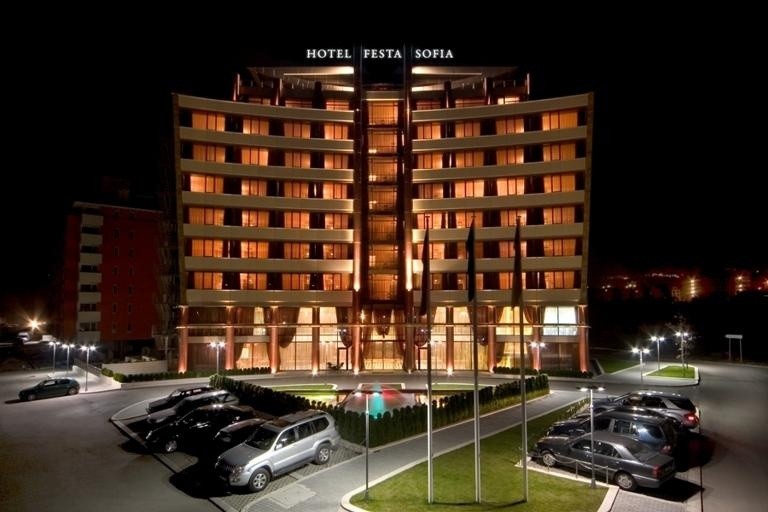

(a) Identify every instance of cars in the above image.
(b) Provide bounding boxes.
[528,390,699,492]
[144,385,341,493]
[19,379,79,401]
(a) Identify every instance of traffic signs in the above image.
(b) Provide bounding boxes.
[725,335,742,339]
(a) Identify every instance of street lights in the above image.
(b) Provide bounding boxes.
[675,332,687,367]
[631,347,649,384]
[62,344,74,376]
[650,336,664,370]
[210,342,225,374]
[81,345,95,391]
[48,342,60,375]
[530,342,544,372]
[351,390,382,503]
